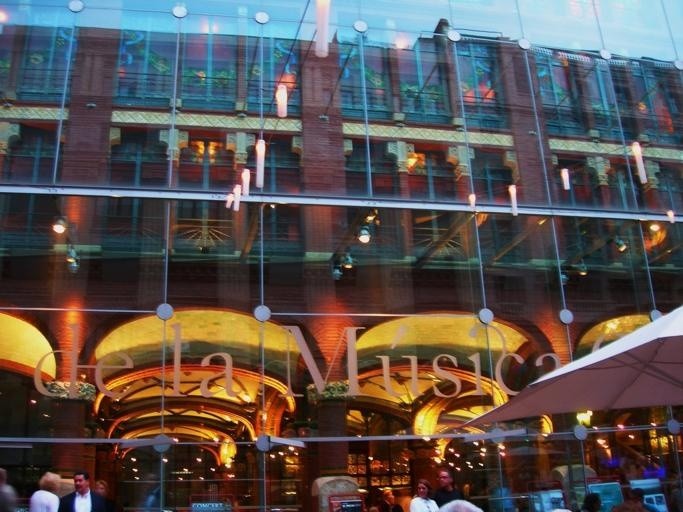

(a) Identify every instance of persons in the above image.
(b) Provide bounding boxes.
[0,465,126,512]
[579,491,602,512]
[432,468,462,507]
[409,480,439,512]
[611,488,656,512]
[137,473,165,512]
[380,489,400,512]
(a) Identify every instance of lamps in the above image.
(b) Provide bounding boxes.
[558,236,629,290]
[50,218,81,277]
[328,220,370,283]
